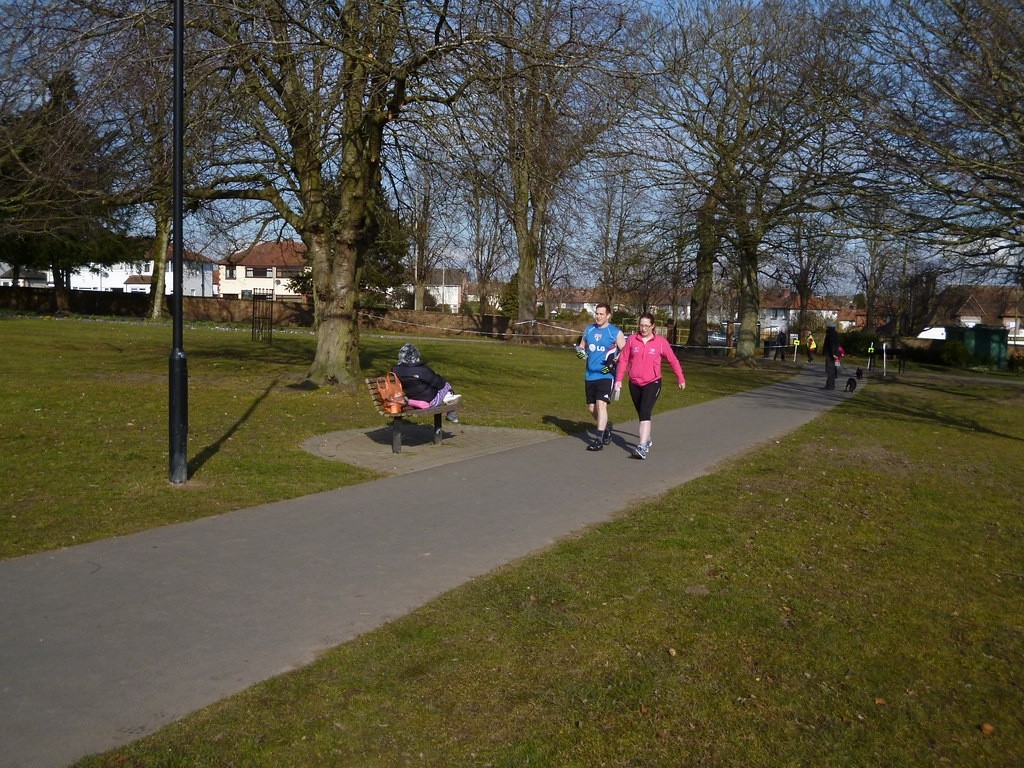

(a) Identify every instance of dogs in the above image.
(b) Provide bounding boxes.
[845,366,864,393]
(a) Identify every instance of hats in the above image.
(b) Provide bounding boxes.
[398,343,421,364]
[806,330,811,334]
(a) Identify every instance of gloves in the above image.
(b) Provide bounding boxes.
[576,348,585,359]
[601,361,614,374]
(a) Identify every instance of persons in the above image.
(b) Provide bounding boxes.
[391,344,458,423]
[576,302,626,451]
[805,331,817,363]
[820,322,844,390]
[774,330,787,361]
[613,313,686,459]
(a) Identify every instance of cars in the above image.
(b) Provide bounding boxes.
[708,330,739,347]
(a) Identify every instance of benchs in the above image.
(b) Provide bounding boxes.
[364,374,460,453]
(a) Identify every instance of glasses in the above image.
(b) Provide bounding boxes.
[639,323,653,328]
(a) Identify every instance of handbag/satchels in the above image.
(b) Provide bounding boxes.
[376,372,408,414]
[835,359,841,367]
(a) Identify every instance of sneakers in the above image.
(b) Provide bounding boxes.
[442,389,462,405]
[587,440,603,451]
[446,411,459,422]
[603,422,614,445]
[646,436,652,452]
[632,444,646,459]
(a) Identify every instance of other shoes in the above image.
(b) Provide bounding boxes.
[811,360,815,364]
[805,360,811,364]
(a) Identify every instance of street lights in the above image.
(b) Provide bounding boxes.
[442,266,446,303]
[414,185,419,286]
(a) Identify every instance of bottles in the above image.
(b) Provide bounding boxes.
[615,384,620,401]
[602,367,610,373]
[574,344,588,360]
